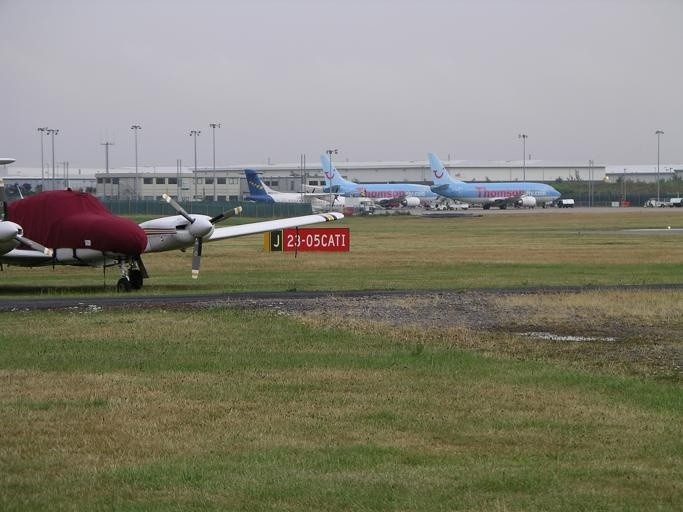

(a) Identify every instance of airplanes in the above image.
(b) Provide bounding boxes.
[0,158,345,294]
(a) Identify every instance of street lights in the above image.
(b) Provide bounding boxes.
[37,127,59,191]
[518,133,529,182]
[209,123,221,203]
[326,149,337,213]
[99,141,115,174]
[130,124,142,173]
[655,130,664,201]
[188,129,201,195]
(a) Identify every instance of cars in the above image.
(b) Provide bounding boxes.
[644,198,683,208]
